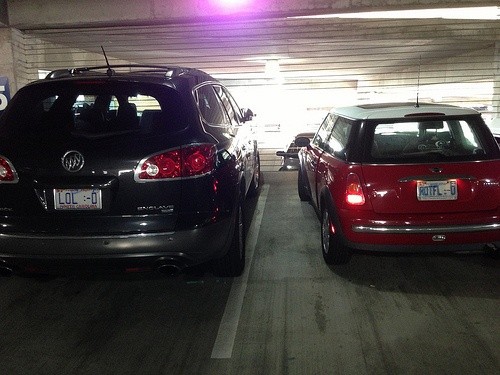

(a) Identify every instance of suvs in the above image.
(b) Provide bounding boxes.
[0,64,261,280]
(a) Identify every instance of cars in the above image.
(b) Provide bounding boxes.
[275,133,319,170]
[294,101,500,266]
